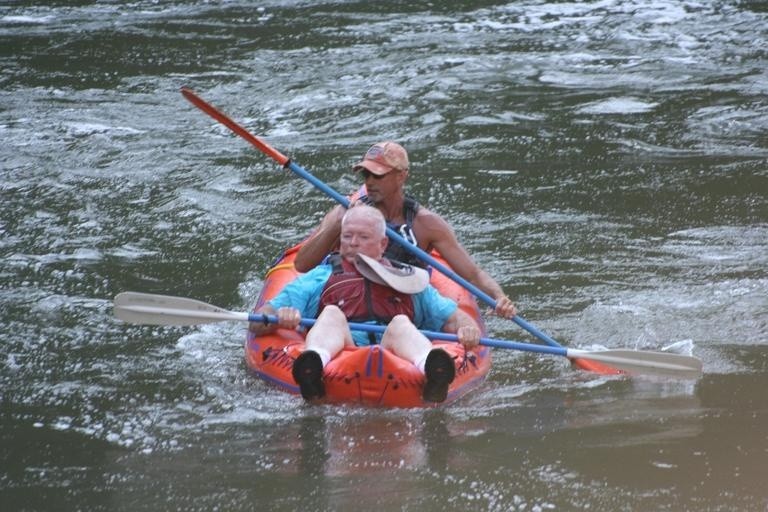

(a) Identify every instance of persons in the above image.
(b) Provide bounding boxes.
[294,141,520,320]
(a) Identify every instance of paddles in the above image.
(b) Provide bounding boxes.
[178,89,635,375]
[114,293,703,380]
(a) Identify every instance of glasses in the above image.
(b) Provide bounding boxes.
[360,170,386,179]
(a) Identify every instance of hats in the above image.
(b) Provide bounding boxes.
[352,140,409,176]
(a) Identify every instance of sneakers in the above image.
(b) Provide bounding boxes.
[421,346,457,404]
[292,349,327,404]
